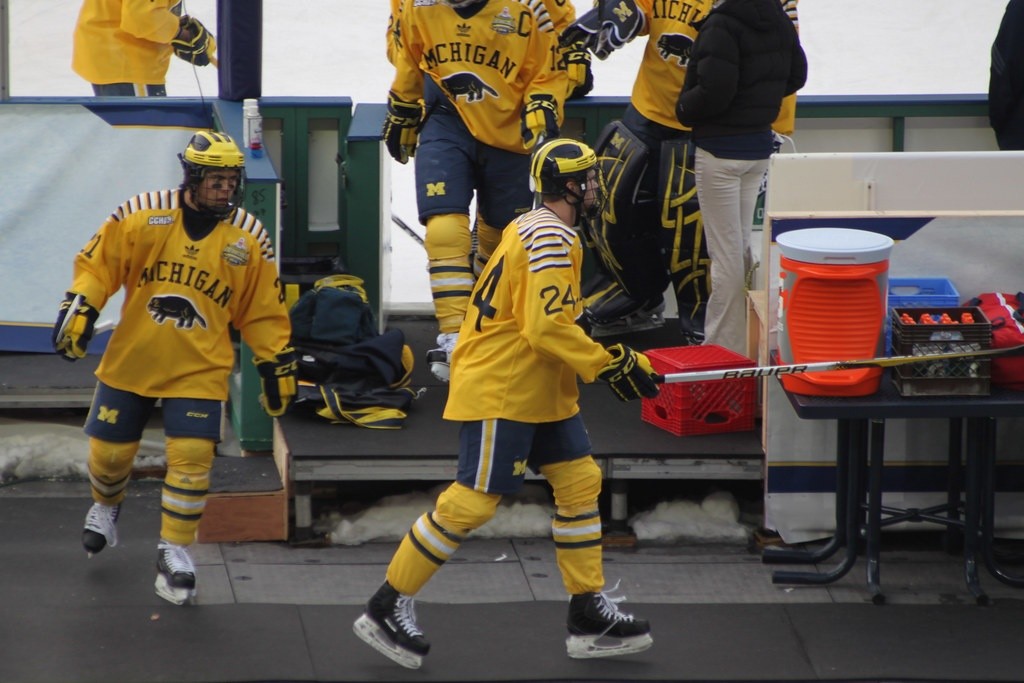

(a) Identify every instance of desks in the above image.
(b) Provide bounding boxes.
[762,349,1024,605]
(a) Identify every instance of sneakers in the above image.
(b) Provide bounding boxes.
[583,289,666,337]
[426,330,464,382]
[153,534,198,608]
[350,574,431,670]
[684,329,705,346]
[563,583,653,660]
[81,501,120,559]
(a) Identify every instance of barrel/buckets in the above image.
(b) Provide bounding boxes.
[775,227,895,397]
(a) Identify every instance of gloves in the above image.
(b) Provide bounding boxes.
[253,348,299,420]
[594,346,659,402]
[52,292,100,363]
[517,99,556,150]
[168,12,217,67]
[560,50,593,101]
[382,91,422,166]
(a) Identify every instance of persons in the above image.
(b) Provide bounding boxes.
[352,138,661,668]
[675,0,808,357]
[560,0,799,341]
[50,132,297,604]
[384,0,593,382]
[70,0,216,96]
[987,0,1024,150]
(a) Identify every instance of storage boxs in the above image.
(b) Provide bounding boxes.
[892,305,992,397]
[641,343,758,437]
[887,276,960,359]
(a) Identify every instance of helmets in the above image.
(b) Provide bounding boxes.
[528,136,608,221]
[178,131,247,221]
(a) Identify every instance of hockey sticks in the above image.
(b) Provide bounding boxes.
[646,331,1022,385]
[208,52,426,248]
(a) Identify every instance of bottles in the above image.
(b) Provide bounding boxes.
[241,97,266,159]
